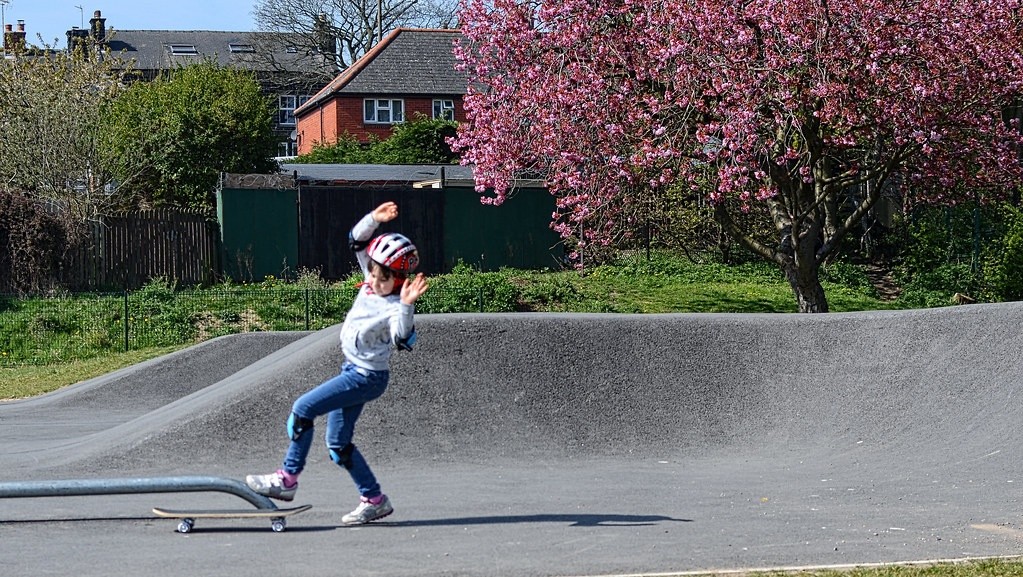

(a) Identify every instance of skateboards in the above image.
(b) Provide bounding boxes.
[152,503,313,534]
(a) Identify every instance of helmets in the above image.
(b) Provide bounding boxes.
[367,232,421,273]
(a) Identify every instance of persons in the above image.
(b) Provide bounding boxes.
[251,199,425,523]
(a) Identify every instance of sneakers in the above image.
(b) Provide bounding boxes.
[245,471,298,502]
[341,495,392,526]
